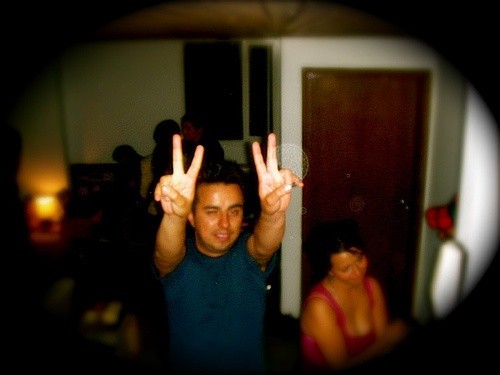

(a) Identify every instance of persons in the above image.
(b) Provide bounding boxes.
[300,236,408,370]
[0,109,305,375]
